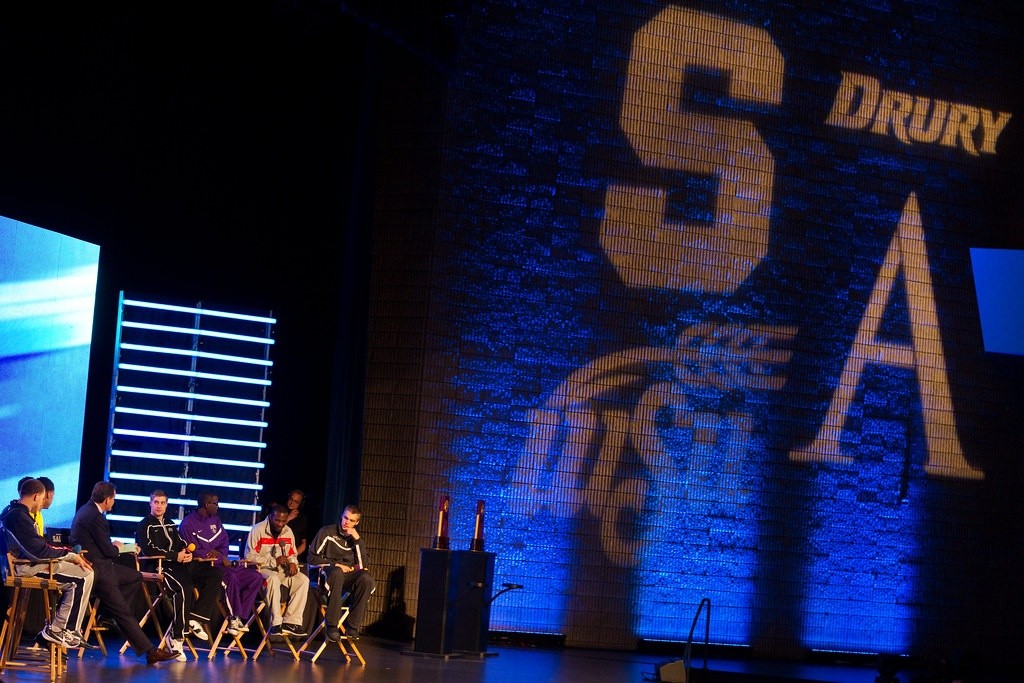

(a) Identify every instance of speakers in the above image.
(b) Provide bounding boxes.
[655,660,686,683]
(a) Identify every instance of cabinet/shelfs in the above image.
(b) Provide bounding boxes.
[413,548,498,656]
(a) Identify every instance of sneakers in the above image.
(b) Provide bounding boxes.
[42,625,80,649]
[324,617,340,643]
[165,632,188,662]
[235,616,249,632]
[226,617,238,634]
[188,620,208,641]
[282,623,309,637]
[269,625,281,635]
[345,623,359,640]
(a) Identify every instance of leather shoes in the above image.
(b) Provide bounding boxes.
[97,614,121,635]
[73,633,94,651]
[34,631,69,661]
[146,648,181,665]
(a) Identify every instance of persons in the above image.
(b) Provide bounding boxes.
[137,490,263,662]
[243,488,374,642]
[0,476,95,649]
[69,481,182,664]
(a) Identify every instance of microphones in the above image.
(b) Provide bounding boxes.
[73,544,82,553]
[185,543,196,554]
[351,565,360,571]
[279,540,287,568]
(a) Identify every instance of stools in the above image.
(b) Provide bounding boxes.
[0,549,367,681]
